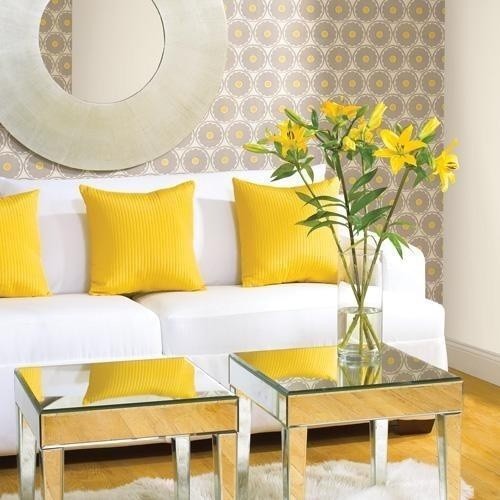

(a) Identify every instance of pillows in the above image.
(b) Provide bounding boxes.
[82,359,197,405]
[0,189,52,297]
[78,180,206,295]
[239,346,339,383]
[17,367,44,403]
[232,177,342,287]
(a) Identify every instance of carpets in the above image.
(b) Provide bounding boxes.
[0,458,474,499]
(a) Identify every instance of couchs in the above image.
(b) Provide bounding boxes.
[0,162,448,457]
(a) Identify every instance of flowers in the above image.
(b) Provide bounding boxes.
[243,102,459,353]
